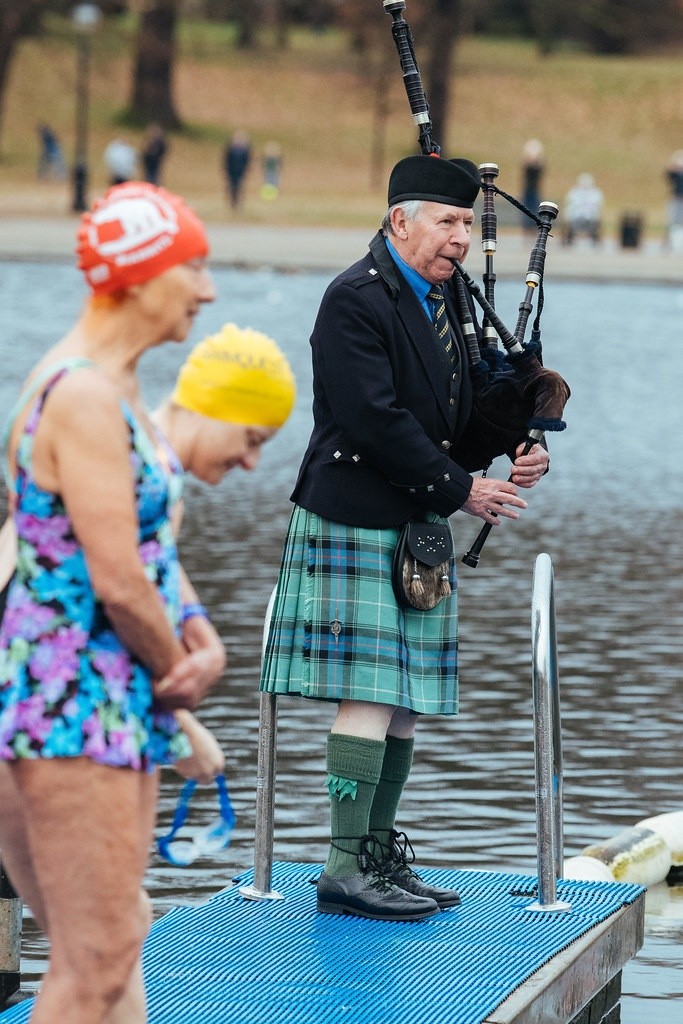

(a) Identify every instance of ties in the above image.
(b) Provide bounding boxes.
[428,284,457,366]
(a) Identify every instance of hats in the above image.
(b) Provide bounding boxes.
[75,181,212,298]
[387,155,482,208]
[171,323,297,428]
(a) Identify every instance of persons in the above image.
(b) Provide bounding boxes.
[25,108,256,211]
[520,142,683,256]
[259,153,551,920]
[0,179,296,1024]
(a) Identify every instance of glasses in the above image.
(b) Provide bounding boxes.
[159,822,230,863]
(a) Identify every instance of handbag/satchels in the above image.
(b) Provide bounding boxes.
[391,519,453,612]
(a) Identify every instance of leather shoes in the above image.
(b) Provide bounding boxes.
[316,833,440,921]
[374,827,462,907]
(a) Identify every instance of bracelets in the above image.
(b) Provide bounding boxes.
[179,603,211,627]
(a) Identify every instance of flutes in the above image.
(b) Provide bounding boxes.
[387,1,571,568]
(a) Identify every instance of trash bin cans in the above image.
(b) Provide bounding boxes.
[621,216,640,246]
